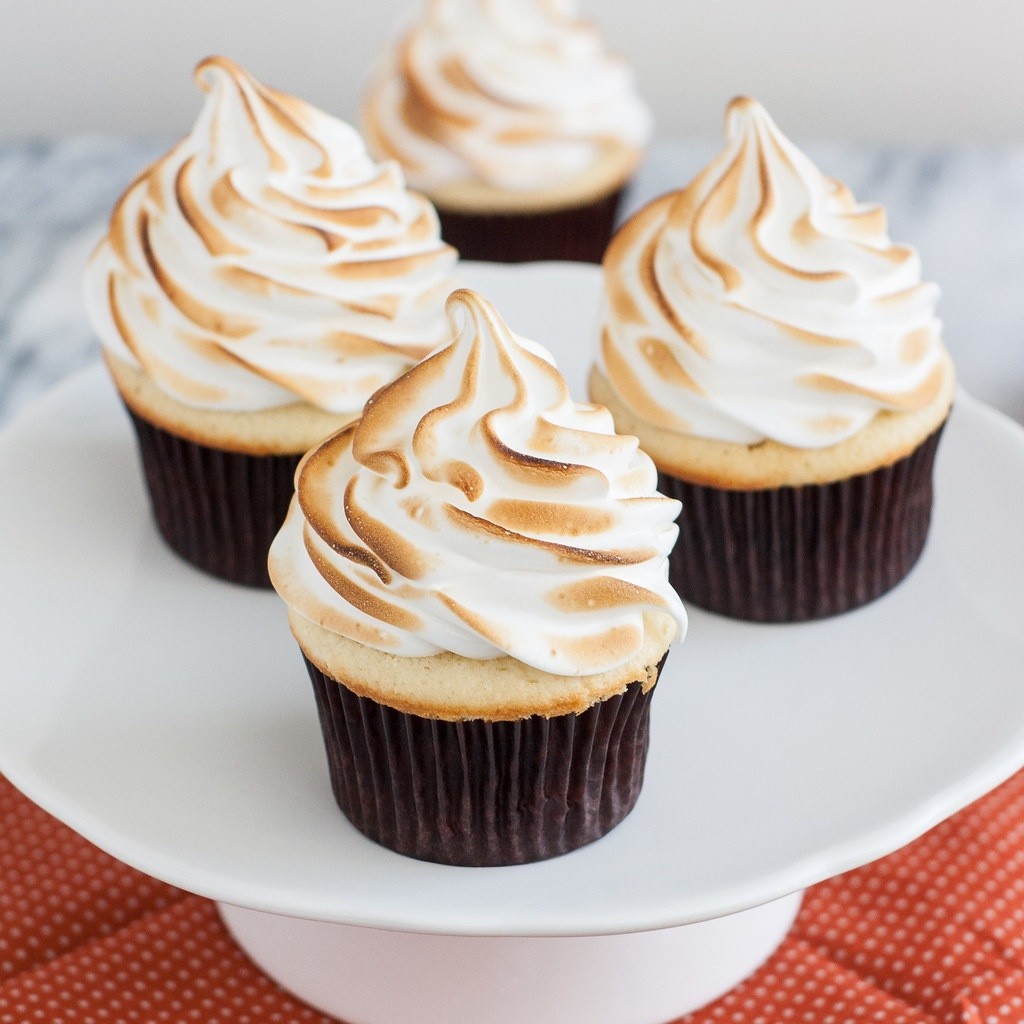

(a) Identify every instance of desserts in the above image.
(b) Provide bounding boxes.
[588,98,957,620]
[85,57,463,591]
[266,291,690,867]
[364,1,652,263]
